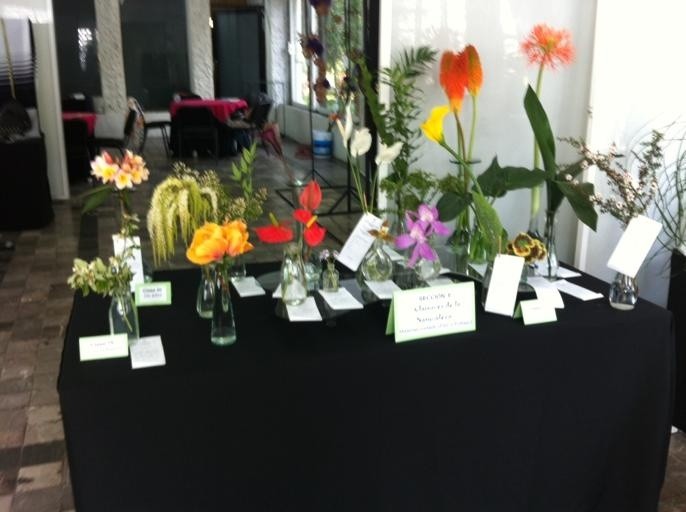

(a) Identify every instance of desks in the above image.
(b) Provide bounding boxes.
[60,109,97,159]
[56,256,676,512]
[170,97,251,160]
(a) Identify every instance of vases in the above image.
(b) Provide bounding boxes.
[411,250,443,282]
[110,230,145,294]
[360,218,395,282]
[322,262,340,293]
[225,250,247,285]
[195,262,216,319]
[281,241,308,307]
[210,259,237,347]
[446,228,472,247]
[109,288,141,348]
[480,263,492,308]
[608,271,640,311]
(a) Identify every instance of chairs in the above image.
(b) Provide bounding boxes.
[81,110,136,190]
[124,95,172,156]
[245,94,285,157]
[172,89,201,101]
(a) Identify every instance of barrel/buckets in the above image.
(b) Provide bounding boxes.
[313,129,332,160]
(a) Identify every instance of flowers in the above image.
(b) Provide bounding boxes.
[418,104,504,263]
[145,160,220,307]
[436,43,483,234]
[298,0,342,108]
[65,210,142,334]
[255,179,327,283]
[318,246,342,291]
[552,127,663,226]
[198,138,268,267]
[72,146,151,234]
[326,68,352,133]
[392,203,455,269]
[520,25,576,222]
[504,230,549,284]
[335,105,405,281]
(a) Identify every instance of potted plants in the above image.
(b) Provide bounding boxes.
[470,81,599,283]
